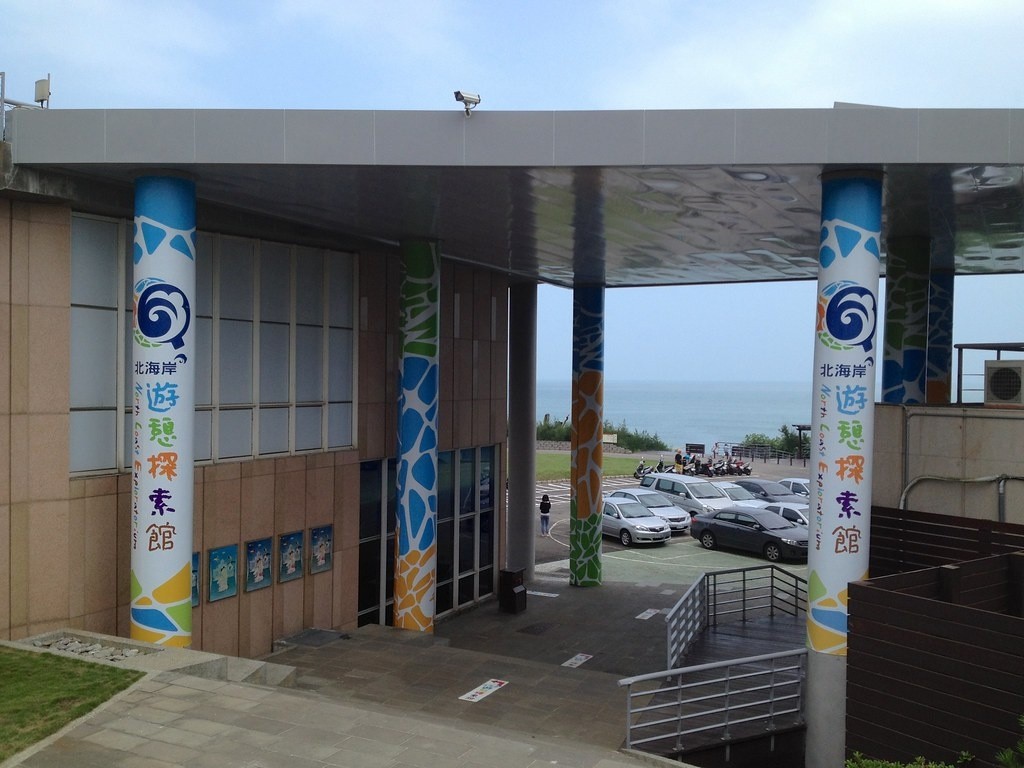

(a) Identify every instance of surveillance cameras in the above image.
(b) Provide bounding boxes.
[454,91,481,109]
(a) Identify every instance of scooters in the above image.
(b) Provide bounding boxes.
[632,453,752,477]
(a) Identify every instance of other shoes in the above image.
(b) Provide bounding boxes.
[547,534,549,536]
[542,534,544,536]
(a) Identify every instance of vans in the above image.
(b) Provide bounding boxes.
[639,472,735,518]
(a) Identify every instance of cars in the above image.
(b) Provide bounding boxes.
[778,478,810,499]
[690,505,808,563]
[601,497,672,547]
[739,479,810,506]
[611,488,690,532]
[710,481,769,509]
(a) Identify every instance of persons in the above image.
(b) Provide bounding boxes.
[540,494,552,537]
[674,449,691,475]
[723,444,729,459]
[713,443,720,459]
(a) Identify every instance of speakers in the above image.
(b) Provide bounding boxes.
[35,79,49,103]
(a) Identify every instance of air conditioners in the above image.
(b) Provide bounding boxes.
[984,360,1024,409]
